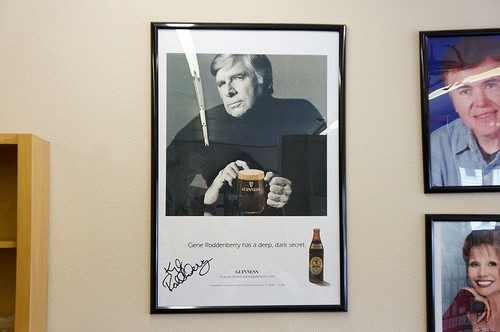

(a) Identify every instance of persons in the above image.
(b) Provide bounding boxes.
[165,54,327,216]
[429,37,500,186]
[442,225,500,332]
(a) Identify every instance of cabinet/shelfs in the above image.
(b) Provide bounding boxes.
[1,132,50,331]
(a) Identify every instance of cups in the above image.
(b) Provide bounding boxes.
[236,170,280,215]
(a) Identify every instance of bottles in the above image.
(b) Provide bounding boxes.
[309,229,324,284]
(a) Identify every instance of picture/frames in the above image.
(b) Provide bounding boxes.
[423,213,499,332]
[150,21,349,314]
[418,26,500,195]
